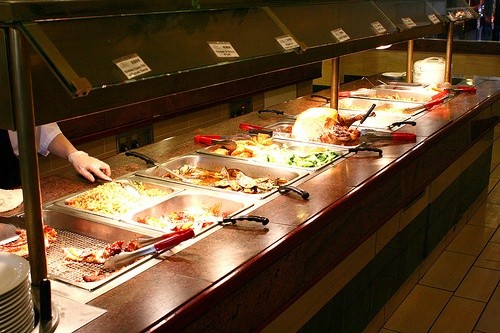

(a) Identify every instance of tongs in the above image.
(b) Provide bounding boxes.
[443,84,477,95]
[193,132,251,152]
[359,127,416,143]
[240,121,294,139]
[103,228,196,273]
[403,94,451,114]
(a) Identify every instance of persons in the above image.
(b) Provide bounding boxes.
[1,122,112,188]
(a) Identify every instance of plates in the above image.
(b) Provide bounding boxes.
[382,71,406,80]
[0,249,36,333]
